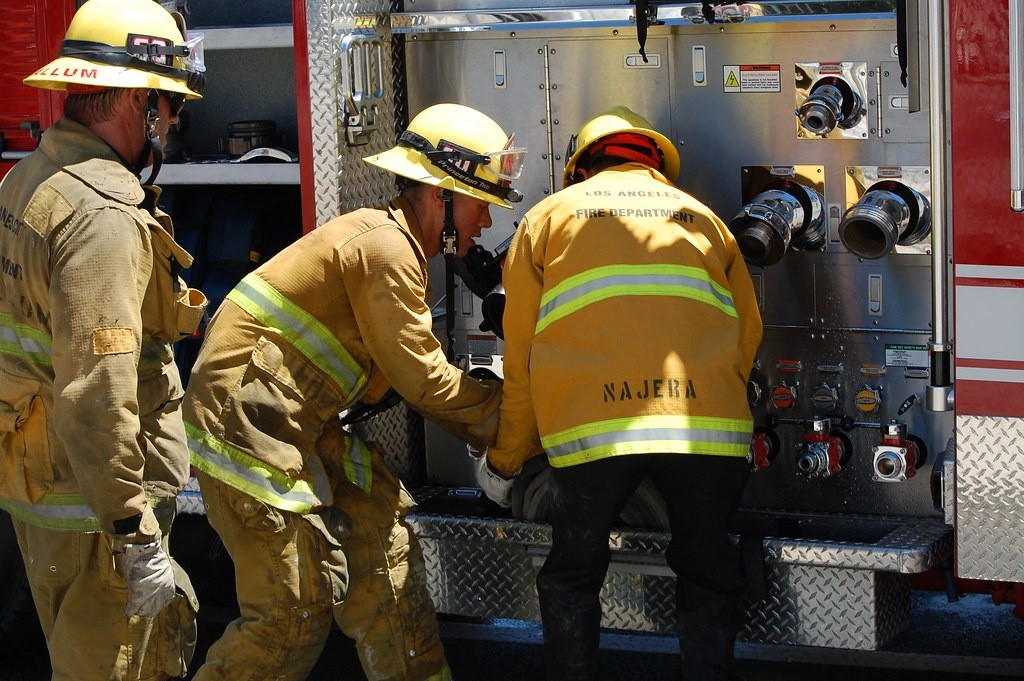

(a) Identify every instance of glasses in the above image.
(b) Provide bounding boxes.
[181,31,207,72]
[158,90,186,118]
[480,147,529,181]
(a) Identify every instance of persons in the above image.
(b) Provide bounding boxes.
[0,0,209,681]
[182,104,519,680]
[474,104,762,681]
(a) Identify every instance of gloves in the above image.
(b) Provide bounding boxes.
[466,444,483,461]
[475,446,523,510]
[122,536,175,617]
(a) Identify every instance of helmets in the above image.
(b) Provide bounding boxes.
[362,103,514,211]
[563,105,681,188]
[23,0,203,99]
